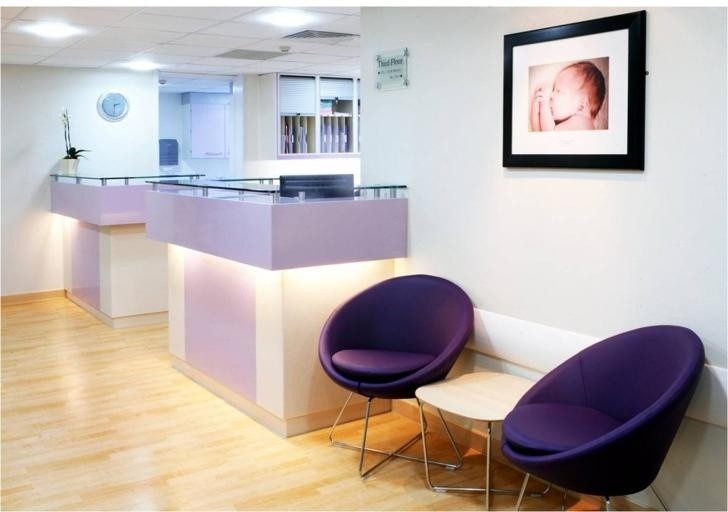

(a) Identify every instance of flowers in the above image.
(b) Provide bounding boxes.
[56,106,92,160]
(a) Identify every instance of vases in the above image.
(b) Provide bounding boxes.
[57,158,81,176]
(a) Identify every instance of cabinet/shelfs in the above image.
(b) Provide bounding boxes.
[256,72,360,160]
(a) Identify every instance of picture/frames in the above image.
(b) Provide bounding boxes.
[502,9,646,171]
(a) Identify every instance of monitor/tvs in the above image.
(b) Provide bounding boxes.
[279,173,355,198]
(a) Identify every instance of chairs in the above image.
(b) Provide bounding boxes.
[317,273,474,478]
[501,325,707,512]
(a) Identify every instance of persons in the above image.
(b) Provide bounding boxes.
[529,61,606,132]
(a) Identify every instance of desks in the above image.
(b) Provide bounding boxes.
[416,370,552,509]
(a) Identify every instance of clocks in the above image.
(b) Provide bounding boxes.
[96,90,129,123]
